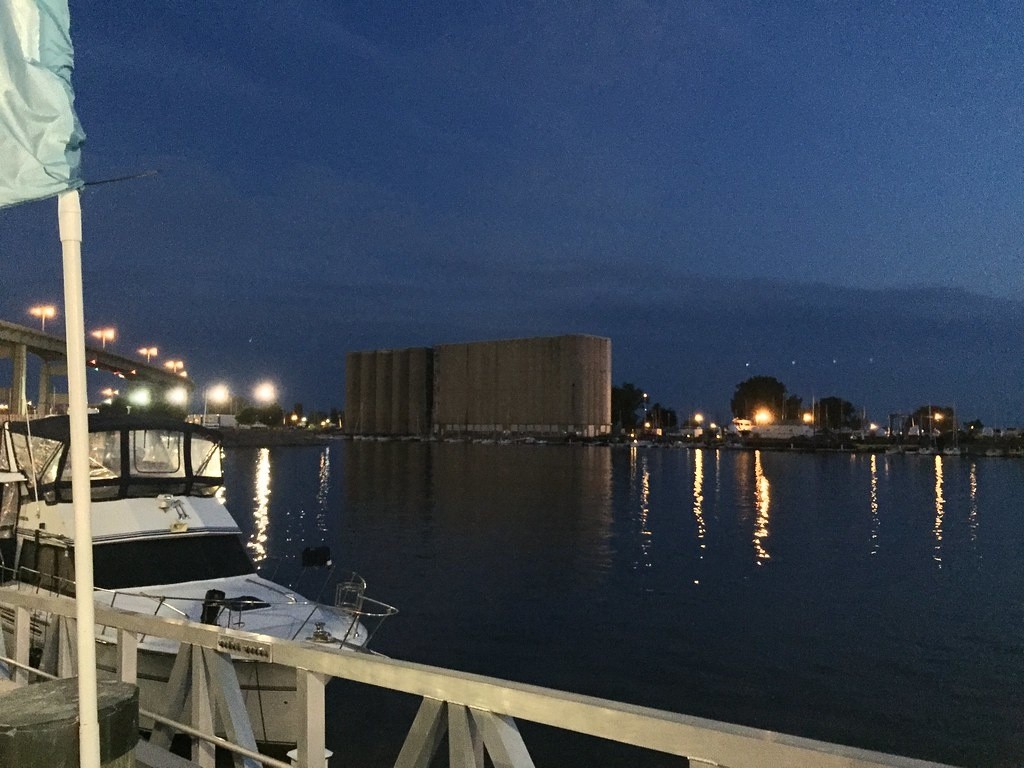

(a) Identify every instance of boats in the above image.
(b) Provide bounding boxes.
[1,399,401,751]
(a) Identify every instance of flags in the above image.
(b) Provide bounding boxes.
[0,0,81,206]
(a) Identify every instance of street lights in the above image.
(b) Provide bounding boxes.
[138,347,158,366]
[164,360,184,376]
[92,327,115,352]
[31,305,56,332]
